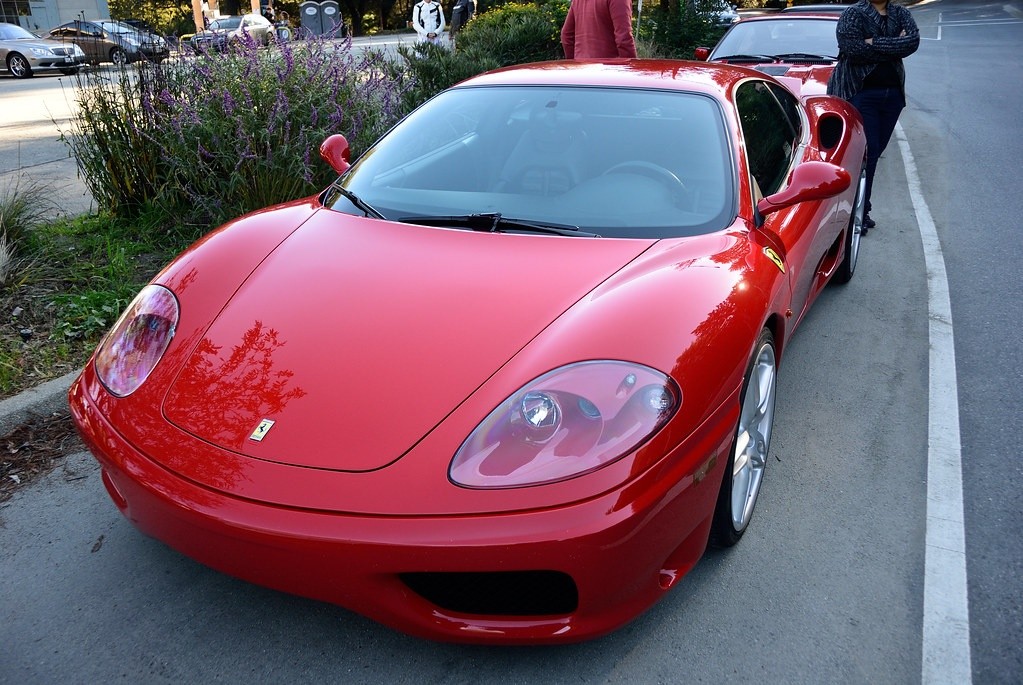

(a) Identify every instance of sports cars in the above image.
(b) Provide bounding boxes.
[66,57,870,649]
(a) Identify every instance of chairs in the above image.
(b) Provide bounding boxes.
[495,103,589,192]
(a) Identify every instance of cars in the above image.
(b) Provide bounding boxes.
[40,19,170,67]
[0,22,86,79]
[189,13,276,53]
[692,2,852,98]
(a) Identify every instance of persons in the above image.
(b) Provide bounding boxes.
[264,8,275,24]
[828,0,920,237]
[449,0,477,39]
[651,122,763,215]
[561,0,639,60]
[412,0,446,61]
